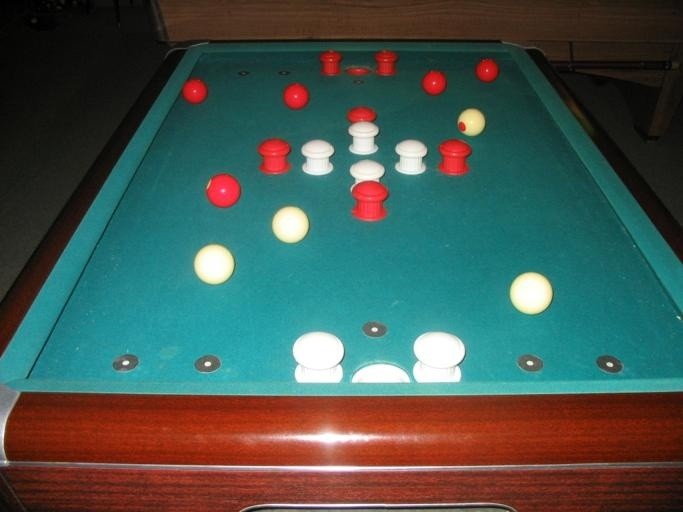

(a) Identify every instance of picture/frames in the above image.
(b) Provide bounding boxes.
[0,39,683,512]
